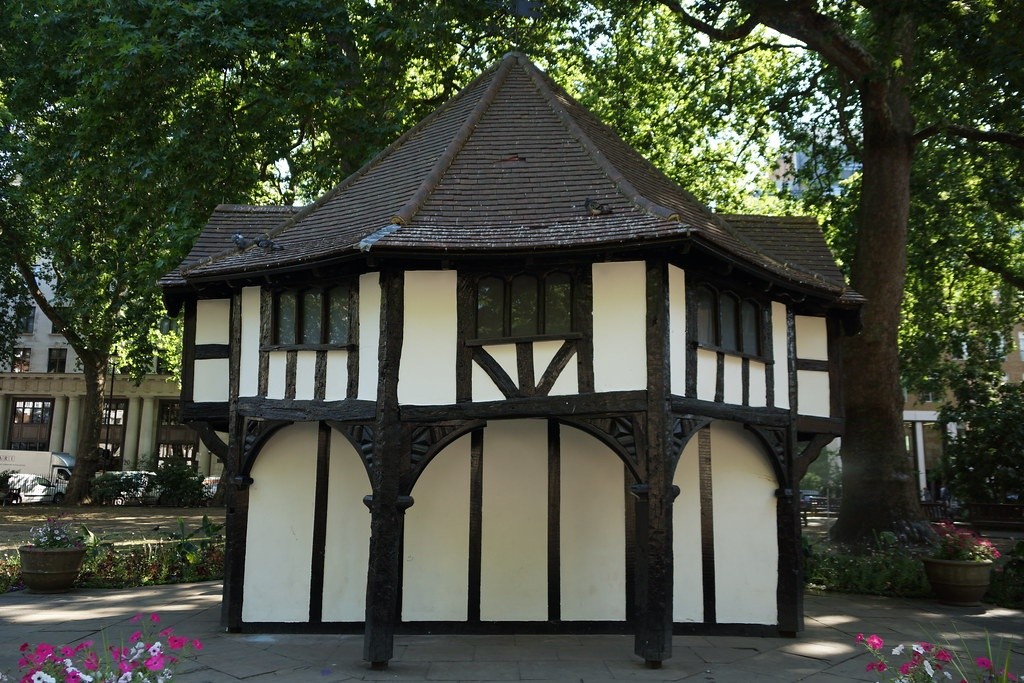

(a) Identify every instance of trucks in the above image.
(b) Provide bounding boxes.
[0,449,78,490]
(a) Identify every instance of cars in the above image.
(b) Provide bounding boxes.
[7,472,67,505]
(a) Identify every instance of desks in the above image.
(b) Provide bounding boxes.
[800,508,812,527]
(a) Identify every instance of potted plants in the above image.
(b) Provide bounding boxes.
[0,470,18,497]
[88,473,158,506]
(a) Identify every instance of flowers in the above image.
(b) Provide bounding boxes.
[926,519,1004,573]
[26,511,87,551]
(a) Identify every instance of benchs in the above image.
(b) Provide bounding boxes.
[919,501,947,522]
[967,501,1024,536]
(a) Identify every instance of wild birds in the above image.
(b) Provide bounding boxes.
[583,195,612,216]
[231,231,248,251]
[252,236,284,251]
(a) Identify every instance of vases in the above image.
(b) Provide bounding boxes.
[18,545,89,594]
[920,556,994,607]
